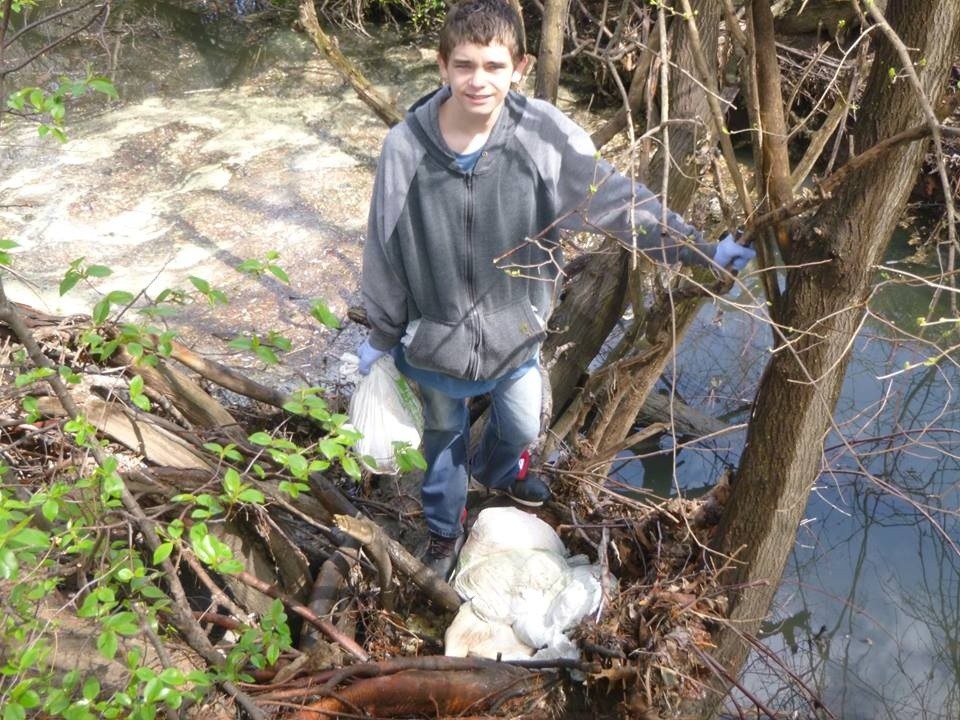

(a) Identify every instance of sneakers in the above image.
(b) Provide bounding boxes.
[421,509,466,585]
[471,471,552,507]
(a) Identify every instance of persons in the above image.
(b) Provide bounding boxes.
[354,1,756,577]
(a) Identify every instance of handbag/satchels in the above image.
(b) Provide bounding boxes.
[348,354,424,477]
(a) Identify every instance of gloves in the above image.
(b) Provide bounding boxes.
[712,233,757,271]
[356,334,389,374]
